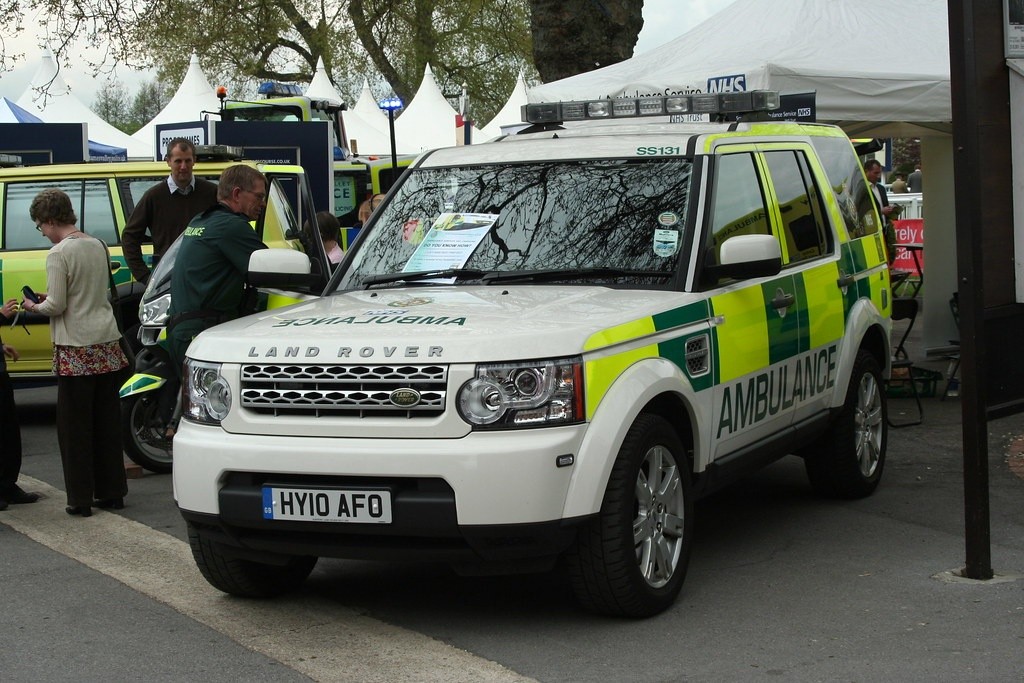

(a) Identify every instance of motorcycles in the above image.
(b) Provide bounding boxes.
[118,228,323,475]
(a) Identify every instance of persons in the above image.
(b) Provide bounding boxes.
[22,188,128,516]
[892,164,922,193]
[855,160,892,228]
[304,211,346,264]
[166,165,270,367]
[358,194,386,224]
[0,298,39,511]
[121,137,218,286]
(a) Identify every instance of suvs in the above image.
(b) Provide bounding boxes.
[0,157,332,390]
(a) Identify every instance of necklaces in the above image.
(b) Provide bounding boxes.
[59,230,80,243]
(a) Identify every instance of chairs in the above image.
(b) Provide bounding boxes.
[890,269,913,298]
[881,300,925,428]
[941,292,960,402]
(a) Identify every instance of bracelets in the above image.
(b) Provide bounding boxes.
[31,303,35,313]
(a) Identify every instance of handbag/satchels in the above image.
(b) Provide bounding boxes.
[118,333,135,391]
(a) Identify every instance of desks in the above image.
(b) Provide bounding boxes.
[892,242,923,297]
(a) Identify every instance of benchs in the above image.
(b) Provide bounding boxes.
[900,278,920,297]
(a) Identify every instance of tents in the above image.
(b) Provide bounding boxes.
[281,54,527,154]
[0,41,224,163]
[527,0,952,140]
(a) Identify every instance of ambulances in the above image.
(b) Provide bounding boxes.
[170,90,893,619]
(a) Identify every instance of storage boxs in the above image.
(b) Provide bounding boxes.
[886,367,938,398]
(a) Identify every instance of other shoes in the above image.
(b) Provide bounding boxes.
[93,499,124,511]
[66,504,92,517]
[1,482,39,510]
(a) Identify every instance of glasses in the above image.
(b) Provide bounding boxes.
[36,220,43,231]
[242,188,266,202]
